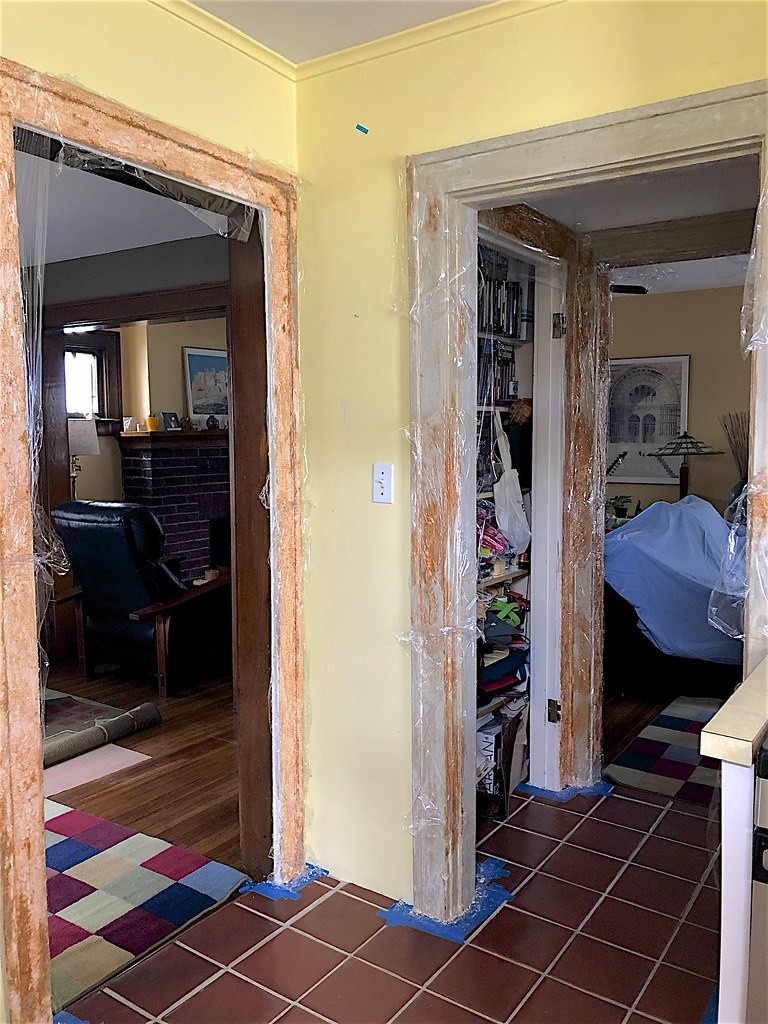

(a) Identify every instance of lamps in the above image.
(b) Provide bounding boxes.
[67,417,100,499]
[646,431,726,500]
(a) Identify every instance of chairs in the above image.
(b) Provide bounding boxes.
[50,500,229,698]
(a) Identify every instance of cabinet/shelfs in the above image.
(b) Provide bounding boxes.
[477,333,532,717]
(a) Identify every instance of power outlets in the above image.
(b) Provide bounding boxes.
[373,464,393,503]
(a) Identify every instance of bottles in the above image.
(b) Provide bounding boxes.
[205,414,219,430]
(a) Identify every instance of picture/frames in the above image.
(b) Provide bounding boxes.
[161,412,181,431]
[181,346,229,429]
[608,354,691,487]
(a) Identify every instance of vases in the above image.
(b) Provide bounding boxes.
[206,415,219,429]
[728,477,747,526]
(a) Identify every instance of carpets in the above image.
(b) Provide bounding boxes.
[43,695,251,1011]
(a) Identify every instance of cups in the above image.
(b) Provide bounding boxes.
[614,507,628,519]
[146,417,160,431]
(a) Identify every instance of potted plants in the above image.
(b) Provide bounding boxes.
[148,413,159,430]
[606,495,633,518]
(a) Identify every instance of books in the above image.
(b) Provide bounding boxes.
[477,279,522,338]
[477,340,516,404]
